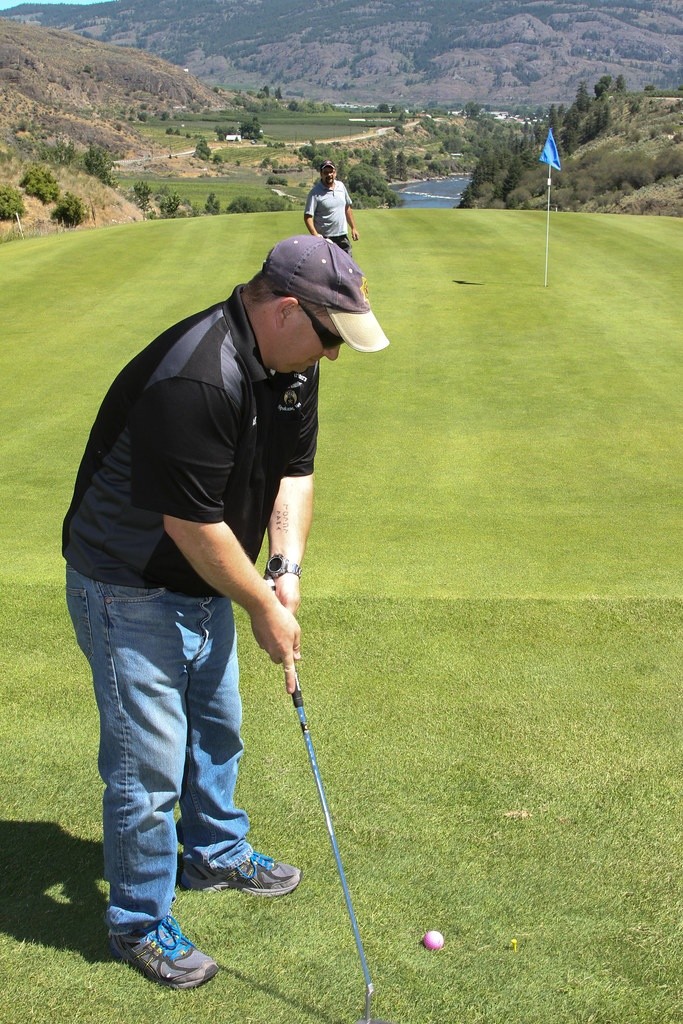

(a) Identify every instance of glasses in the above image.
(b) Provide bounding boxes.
[322,171,335,175]
[297,302,344,349]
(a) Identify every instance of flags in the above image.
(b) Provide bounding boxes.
[539,128,562,172]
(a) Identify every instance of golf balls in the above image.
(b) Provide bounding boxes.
[423,931,444,950]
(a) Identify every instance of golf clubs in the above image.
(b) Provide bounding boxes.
[261,572,387,1024]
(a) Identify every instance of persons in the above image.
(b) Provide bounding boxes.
[304,160,359,258]
[61,237,390,990]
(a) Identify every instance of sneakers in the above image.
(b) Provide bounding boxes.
[181,852,302,897]
[108,915,219,990]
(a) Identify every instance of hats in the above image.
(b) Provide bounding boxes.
[320,160,335,170]
[262,235,390,353]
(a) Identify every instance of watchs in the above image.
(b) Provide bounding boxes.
[265,553,302,578]
